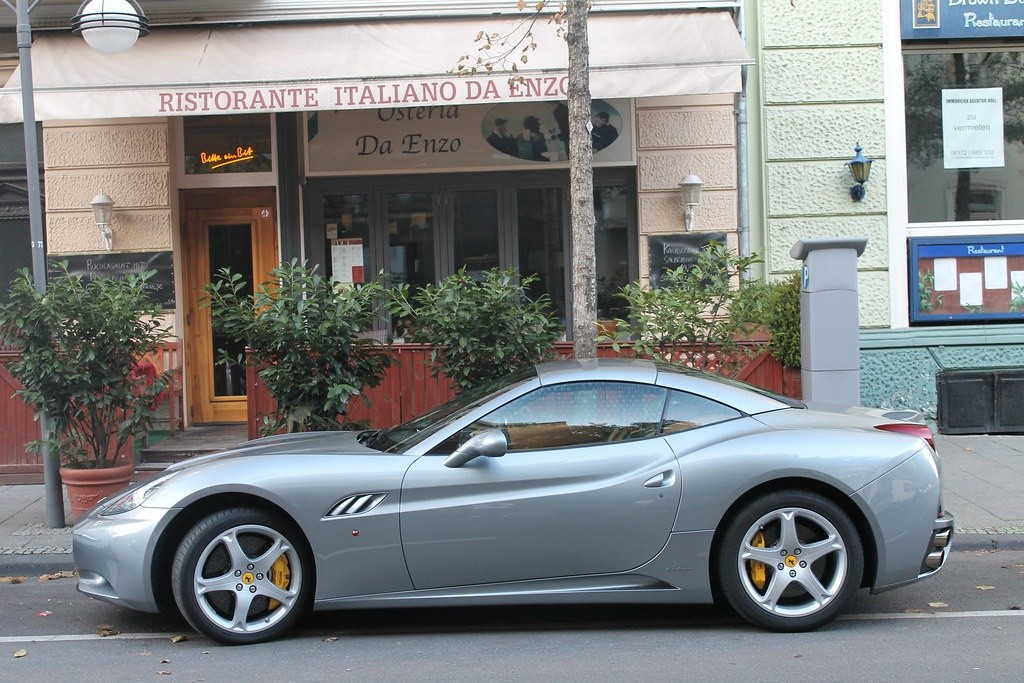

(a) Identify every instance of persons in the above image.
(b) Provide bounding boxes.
[487,118,519,157]
[516,115,550,161]
[545,101,569,160]
[591,112,618,152]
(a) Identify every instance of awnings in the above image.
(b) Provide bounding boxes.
[1,11,756,126]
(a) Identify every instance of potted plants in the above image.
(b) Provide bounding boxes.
[0,255,178,524]
[730,271,803,341]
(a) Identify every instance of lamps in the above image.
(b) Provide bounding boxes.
[70,0,150,54]
[677,170,704,232]
[89,190,116,251]
[843,142,872,201]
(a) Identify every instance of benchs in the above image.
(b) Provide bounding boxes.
[130,339,184,448]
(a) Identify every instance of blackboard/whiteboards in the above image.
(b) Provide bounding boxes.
[45,252,176,315]
[648,231,730,294]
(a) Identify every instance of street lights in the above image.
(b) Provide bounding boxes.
[2,0,150,529]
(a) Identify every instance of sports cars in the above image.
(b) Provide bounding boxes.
[72,356,955,646]
